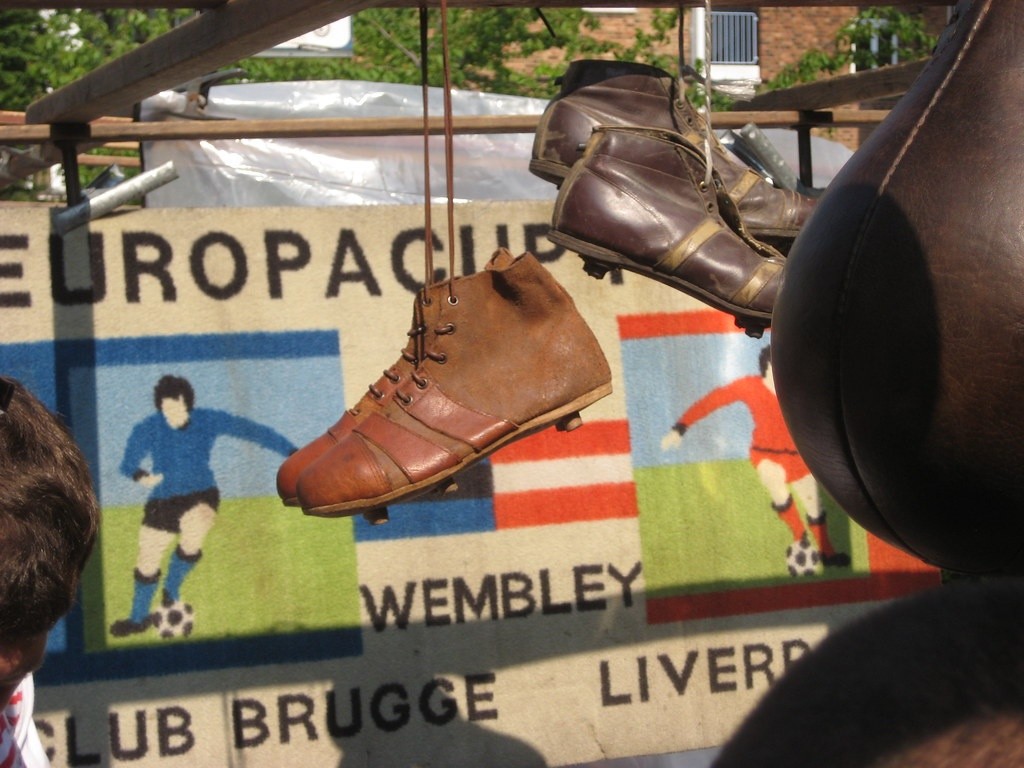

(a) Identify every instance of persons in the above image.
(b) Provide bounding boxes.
[0,374,99,768]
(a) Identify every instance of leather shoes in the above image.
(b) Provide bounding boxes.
[275,246,611,526]
[529,58,821,339]
